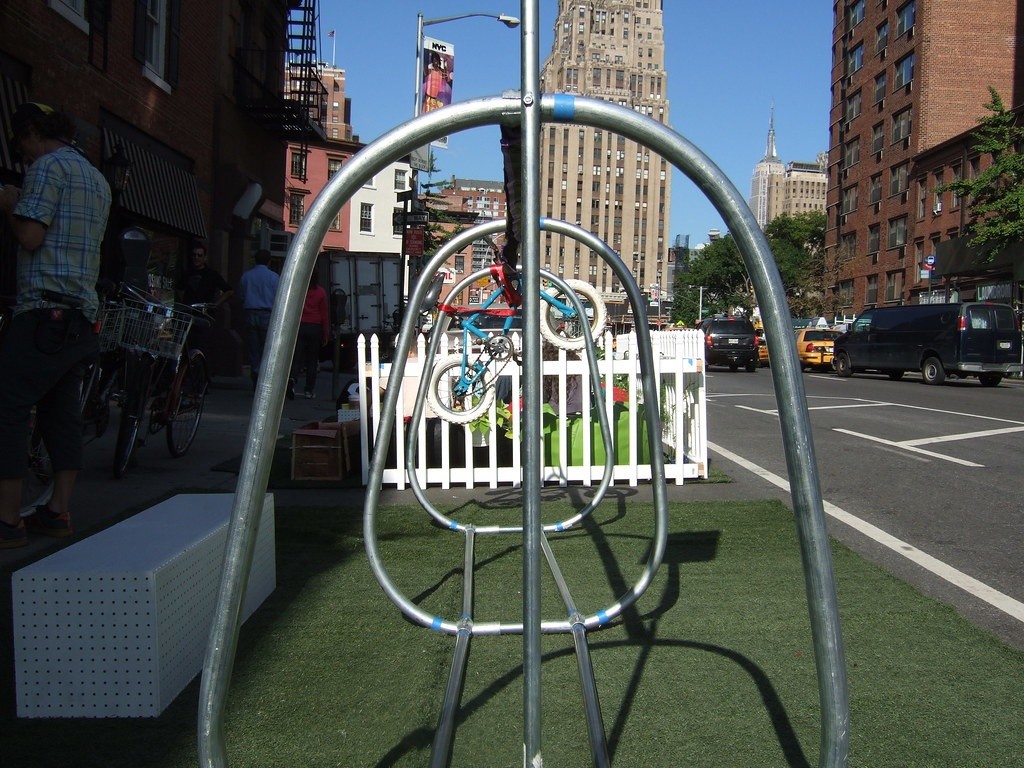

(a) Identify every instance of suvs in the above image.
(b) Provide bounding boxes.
[695,316,759,372]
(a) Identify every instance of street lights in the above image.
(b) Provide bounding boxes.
[404,11,523,320]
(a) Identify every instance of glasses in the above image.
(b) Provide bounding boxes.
[192,252,202,257]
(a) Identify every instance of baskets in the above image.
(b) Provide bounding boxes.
[98,299,193,360]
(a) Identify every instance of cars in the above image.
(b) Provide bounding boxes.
[795,328,845,372]
[757,337,770,368]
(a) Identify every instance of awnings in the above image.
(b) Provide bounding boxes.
[102,127,209,238]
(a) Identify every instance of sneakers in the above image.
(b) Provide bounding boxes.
[24,505,75,537]
[0,515,28,549]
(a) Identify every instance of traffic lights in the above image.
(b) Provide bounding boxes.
[750,286,756,300]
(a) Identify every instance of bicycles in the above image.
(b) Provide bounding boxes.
[420,229,607,428]
[7,281,221,518]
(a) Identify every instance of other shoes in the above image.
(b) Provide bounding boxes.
[183,400,198,412]
[304,390,314,398]
[286,390,295,400]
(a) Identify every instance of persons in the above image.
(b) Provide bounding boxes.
[0,103,113,548]
[286,268,329,401]
[422,51,442,106]
[239,250,280,390]
[496,342,596,415]
[335,336,427,416]
[175,245,235,392]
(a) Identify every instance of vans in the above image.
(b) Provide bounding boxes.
[832,302,1024,388]
